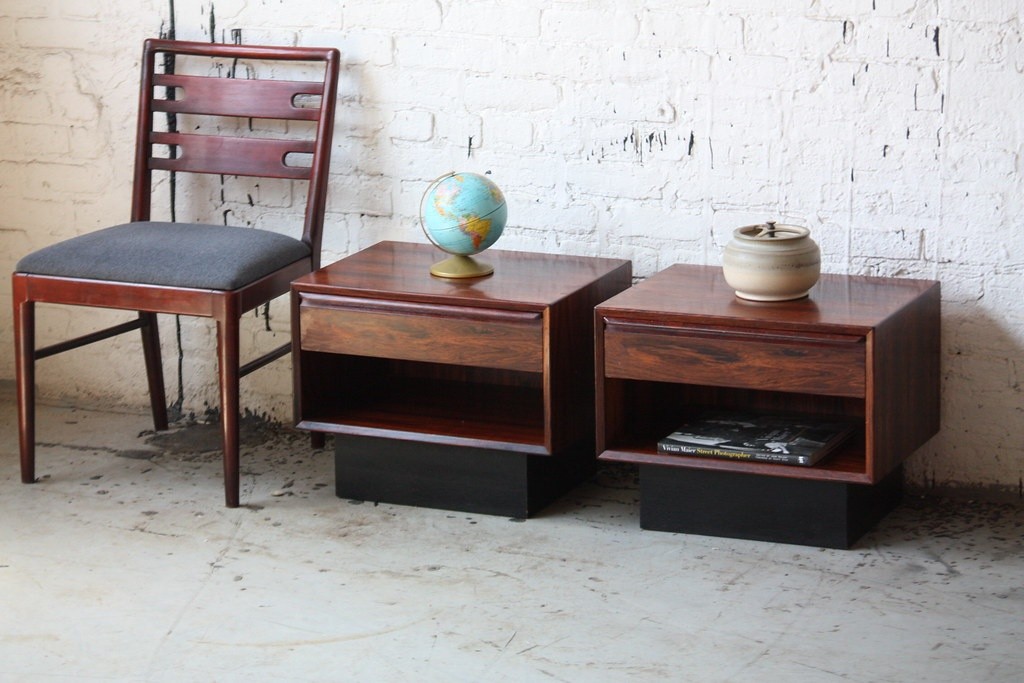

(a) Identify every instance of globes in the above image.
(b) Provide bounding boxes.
[419,170,508,279]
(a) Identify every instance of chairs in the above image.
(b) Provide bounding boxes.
[12,39,341,507]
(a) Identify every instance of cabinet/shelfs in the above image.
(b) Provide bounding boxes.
[290,239,632,517]
[594,262,941,550]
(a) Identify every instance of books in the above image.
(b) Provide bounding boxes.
[657,414,850,467]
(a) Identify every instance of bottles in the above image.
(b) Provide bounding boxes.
[723,221,821,301]
